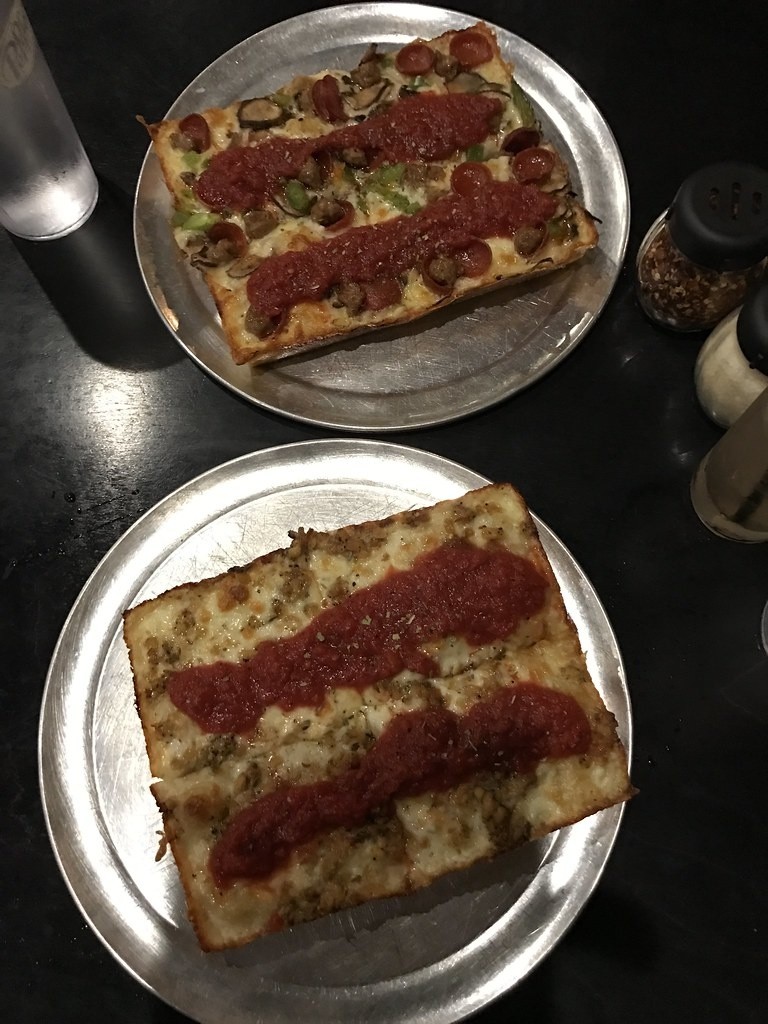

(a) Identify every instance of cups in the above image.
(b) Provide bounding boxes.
[0,0,100,242]
[689,387,768,544]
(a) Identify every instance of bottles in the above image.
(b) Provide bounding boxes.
[635,165,768,333]
[693,285,768,430]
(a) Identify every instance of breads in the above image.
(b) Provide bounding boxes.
[117,482,639,951]
[136,19,602,368]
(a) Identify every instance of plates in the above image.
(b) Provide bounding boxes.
[132,1,633,433]
[36,438,633,1024]
